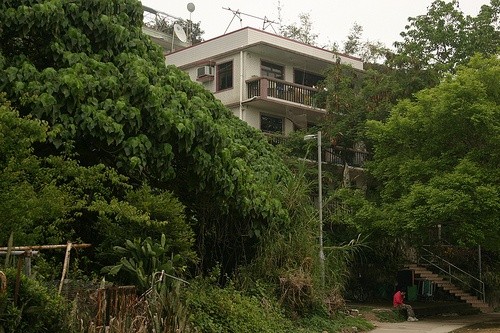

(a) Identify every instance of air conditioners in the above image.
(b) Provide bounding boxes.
[196,65,214,78]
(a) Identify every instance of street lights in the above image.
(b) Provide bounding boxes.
[303,130,325,289]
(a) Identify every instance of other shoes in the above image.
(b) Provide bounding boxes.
[407,316,419,322]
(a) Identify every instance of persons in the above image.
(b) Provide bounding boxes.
[393,288,419,322]
